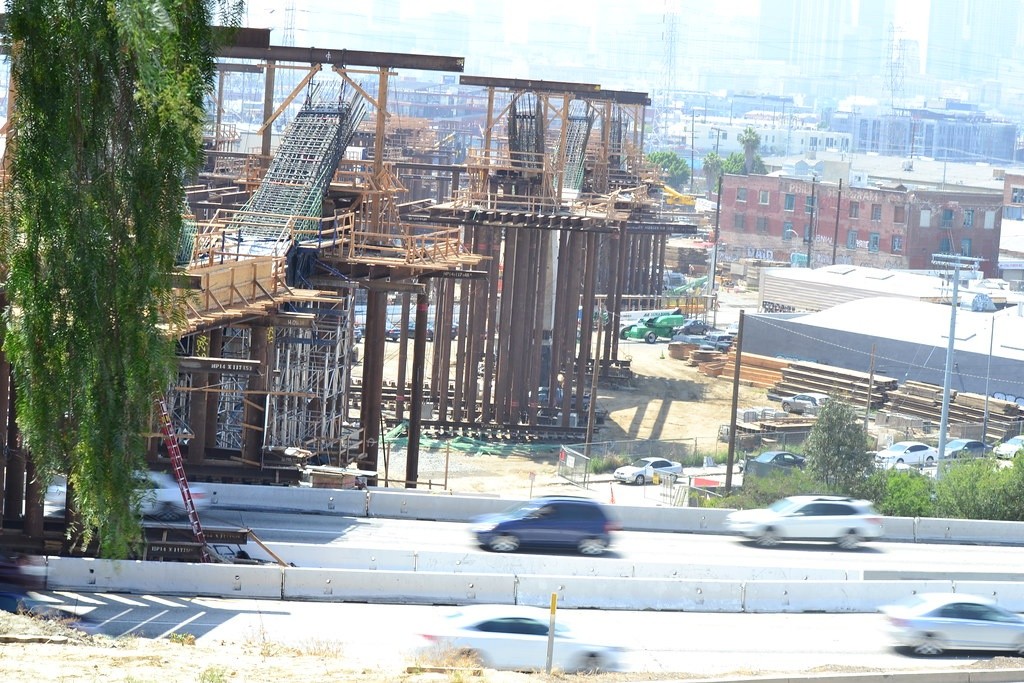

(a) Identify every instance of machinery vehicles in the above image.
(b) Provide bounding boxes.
[658,180,696,212]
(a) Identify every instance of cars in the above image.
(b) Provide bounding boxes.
[451,322,459,340]
[361,321,401,343]
[944,439,994,460]
[408,322,434,341]
[613,457,682,485]
[751,451,807,473]
[423,606,617,677]
[44,468,212,522]
[724,496,882,551]
[993,435,1024,459]
[353,324,362,343]
[875,441,939,467]
[881,592,1024,657]
[781,393,831,415]
[469,498,615,557]
[617,215,780,353]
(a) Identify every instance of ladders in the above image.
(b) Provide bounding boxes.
[148,377,212,564]
